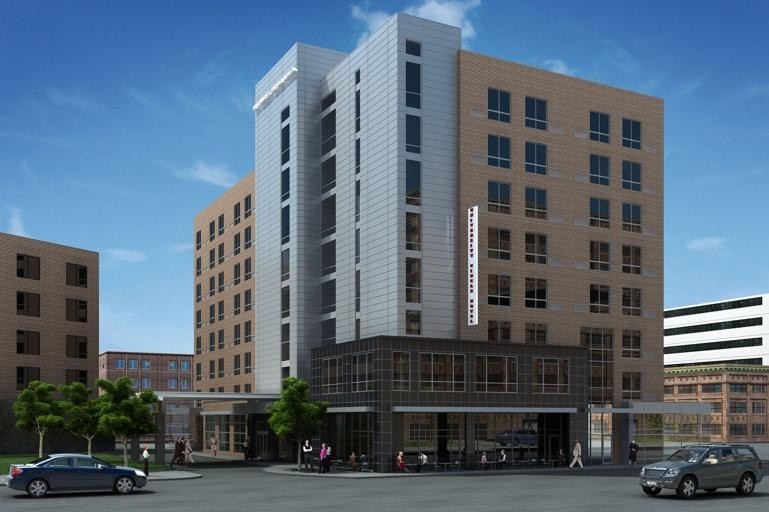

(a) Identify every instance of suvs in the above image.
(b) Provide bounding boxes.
[496,427,536,447]
[636,440,763,501]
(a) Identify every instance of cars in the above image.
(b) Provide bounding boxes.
[3,453,148,498]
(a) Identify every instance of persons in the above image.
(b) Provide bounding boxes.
[481,452,489,470]
[210,433,217,456]
[569,439,583,469]
[629,439,638,466]
[498,450,511,470]
[142,445,150,476]
[169,435,194,467]
[326,444,331,472]
[303,440,315,472]
[318,443,327,473]
[239,434,254,462]
[349,452,357,471]
[359,452,366,471]
[415,451,427,472]
[396,451,411,473]
